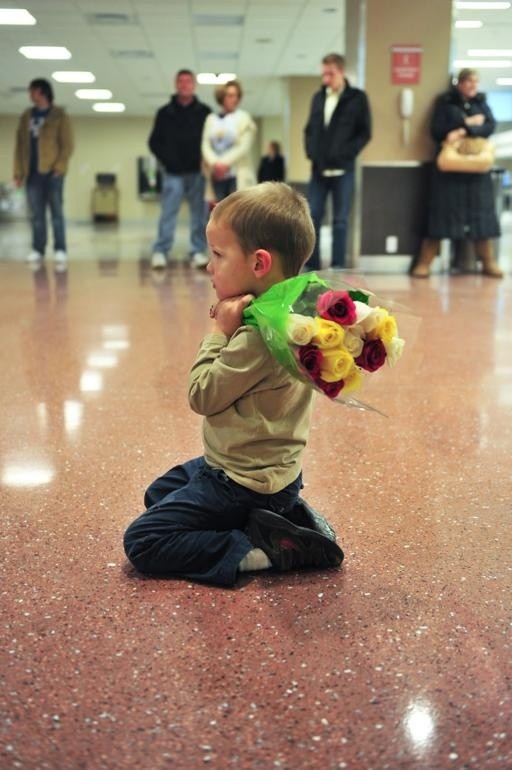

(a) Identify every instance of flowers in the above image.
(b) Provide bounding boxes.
[228,275,404,399]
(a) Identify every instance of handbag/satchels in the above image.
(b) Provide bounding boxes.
[435,136,494,173]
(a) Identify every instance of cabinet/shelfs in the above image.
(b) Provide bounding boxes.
[359,161,448,274]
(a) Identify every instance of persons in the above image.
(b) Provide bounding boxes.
[302,53,371,272]
[200,81,258,201]
[257,142,283,181]
[149,69,211,270]
[125,181,343,591]
[408,69,503,278]
[14,78,74,263]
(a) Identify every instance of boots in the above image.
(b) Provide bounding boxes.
[412,238,437,277]
[475,239,503,277]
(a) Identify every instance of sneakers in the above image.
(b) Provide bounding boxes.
[53,249,67,260]
[190,253,208,269]
[284,494,336,543]
[247,507,344,572]
[152,252,166,268]
[26,250,42,260]
